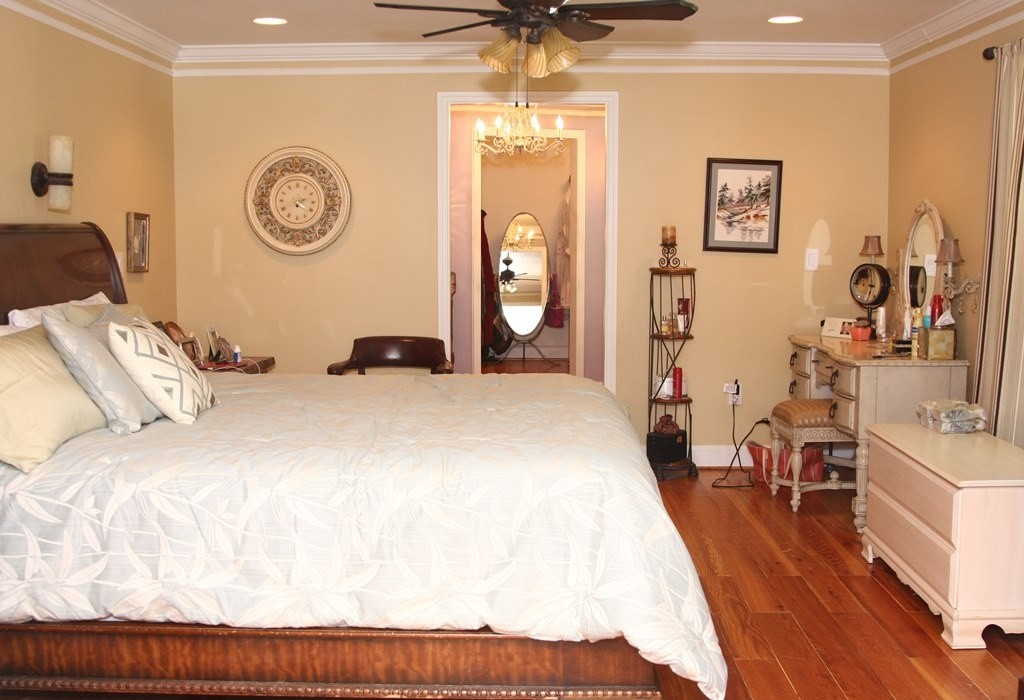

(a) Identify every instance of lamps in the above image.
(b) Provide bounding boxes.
[501,221,534,251]
[472,103,567,159]
[30,134,74,212]
[856,235,886,340]
[480,22,580,107]
[500,279,517,294]
[934,236,965,329]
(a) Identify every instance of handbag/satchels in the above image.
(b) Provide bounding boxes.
[746,439,826,487]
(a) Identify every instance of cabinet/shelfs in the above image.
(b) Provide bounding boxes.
[645,266,699,480]
[787,335,970,534]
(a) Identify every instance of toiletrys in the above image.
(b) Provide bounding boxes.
[677,297,691,334]
[932,293,944,327]
[875,306,886,338]
[661,313,687,334]
[903,303,912,340]
[924,305,932,327]
[912,307,923,357]
[673,367,683,398]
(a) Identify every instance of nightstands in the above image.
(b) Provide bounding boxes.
[198,356,275,374]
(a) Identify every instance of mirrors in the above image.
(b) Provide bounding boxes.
[497,212,561,369]
[897,196,943,341]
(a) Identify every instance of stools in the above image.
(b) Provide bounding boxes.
[770,398,856,512]
[860,424,1024,647]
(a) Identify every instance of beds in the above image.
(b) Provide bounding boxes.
[0,219,729,700]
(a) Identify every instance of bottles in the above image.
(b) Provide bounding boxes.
[234,346,242,364]
[850,320,872,340]
[876,307,887,338]
[672,367,682,398]
[661,316,670,335]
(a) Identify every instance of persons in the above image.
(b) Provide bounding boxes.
[170,328,182,342]
[132,227,147,267]
[184,343,197,360]
[840,322,852,335]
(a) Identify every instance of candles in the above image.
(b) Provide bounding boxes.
[673,367,684,398]
[660,226,676,246]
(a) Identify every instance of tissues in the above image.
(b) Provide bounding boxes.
[917,310,958,361]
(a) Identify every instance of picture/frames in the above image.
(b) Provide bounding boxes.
[166,321,187,344]
[125,211,151,273]
[179,338,202,366]
[207,329,220,359]
[703,156,783,255]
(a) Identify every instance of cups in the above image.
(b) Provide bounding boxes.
[877,333,891,342]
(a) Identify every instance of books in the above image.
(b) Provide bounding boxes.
[821,317,856,339]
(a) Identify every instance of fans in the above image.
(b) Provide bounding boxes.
[498,253,541,284]
[372,1,699,39]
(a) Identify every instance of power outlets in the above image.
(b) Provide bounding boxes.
[729,383,741,405]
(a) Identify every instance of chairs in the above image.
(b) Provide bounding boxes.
[327,336,456,374]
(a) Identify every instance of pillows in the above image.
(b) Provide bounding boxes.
[0,291,221,474]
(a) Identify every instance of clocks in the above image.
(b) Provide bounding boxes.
[852,263,889,339]
[908,265,927,307]
[244,146,352,255]
